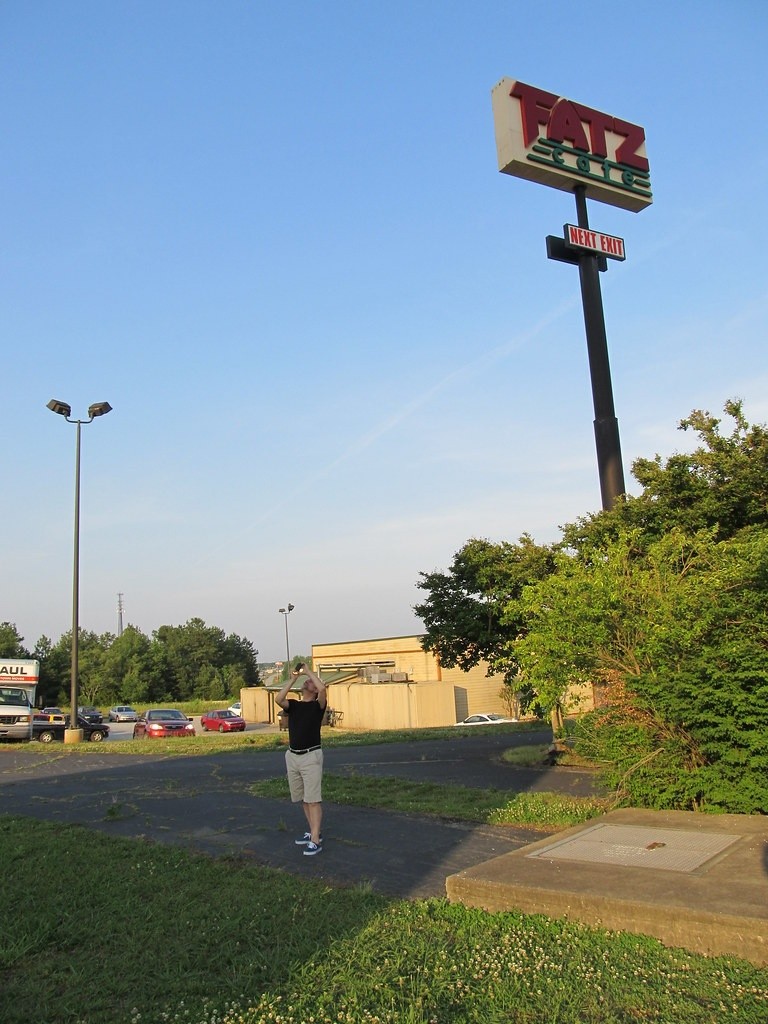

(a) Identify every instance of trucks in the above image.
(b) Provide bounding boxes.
[0,658,45,741]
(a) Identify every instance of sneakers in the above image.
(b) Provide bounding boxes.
[295,832,323,844]
[304,843,323,856]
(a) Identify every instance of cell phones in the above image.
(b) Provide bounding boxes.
[295,663,302,671]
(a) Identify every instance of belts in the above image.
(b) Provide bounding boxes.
[288,746,322,755]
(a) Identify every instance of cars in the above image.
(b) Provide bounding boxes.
[77,706,104,724]
[454,713,517,725]
[40,708,64,714]
[133,708,196,738]
[200,710,246,733]
[108,705,137,722]
[228,702,241,717]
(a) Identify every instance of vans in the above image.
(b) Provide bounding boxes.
[33,713,68,725]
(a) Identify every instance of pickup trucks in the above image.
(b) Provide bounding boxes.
[33,713,110,744]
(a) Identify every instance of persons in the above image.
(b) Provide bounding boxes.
[276,661,327,856]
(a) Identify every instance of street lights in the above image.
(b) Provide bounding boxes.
[47,396,115,732]
[279,603,296,681]
